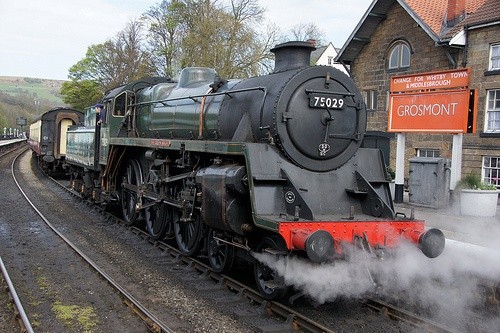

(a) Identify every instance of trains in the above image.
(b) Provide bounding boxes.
[29,40,446,304]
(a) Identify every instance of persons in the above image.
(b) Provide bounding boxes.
[94,104,107,126]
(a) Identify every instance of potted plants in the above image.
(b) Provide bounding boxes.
[453,172,500,216]
